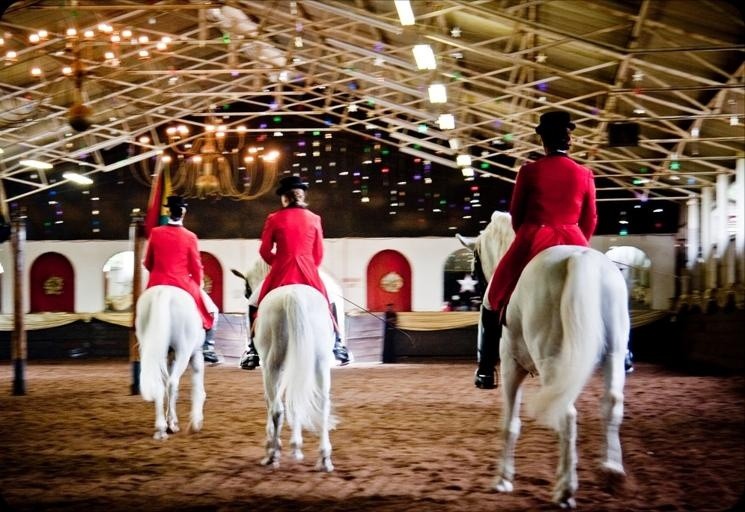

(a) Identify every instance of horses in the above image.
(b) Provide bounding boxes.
[132,282,208,441]
[230,251,338,479]
[450,209,632,510]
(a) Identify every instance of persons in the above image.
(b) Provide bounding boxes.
[474,110,637,388]
[237,175,352,370]
[142,193,221,366]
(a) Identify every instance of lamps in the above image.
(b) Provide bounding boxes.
[0,1,172,131]
[125,113,282,205]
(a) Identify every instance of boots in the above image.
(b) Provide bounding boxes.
[328,302,350,366]
[202,327,219,362]
[475,305,501,390]
[240,304,259,370]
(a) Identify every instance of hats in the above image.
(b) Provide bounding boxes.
[536,111,575,135]
[162,196,188,207]
[276,176,309,196]
